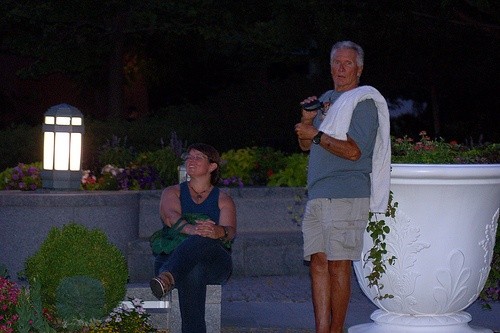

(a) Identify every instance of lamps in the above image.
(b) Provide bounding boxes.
[41,102,85,192]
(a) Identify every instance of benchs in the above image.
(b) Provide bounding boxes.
[122,285,222,333]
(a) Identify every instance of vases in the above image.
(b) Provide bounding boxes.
[0,187,306,276]
[352,163,500,325]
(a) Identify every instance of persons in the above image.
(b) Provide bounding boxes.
[294,42,378,333]
[150,144,238,333]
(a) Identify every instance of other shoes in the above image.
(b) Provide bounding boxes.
[150,278,170,300]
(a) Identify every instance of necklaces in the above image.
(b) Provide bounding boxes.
[190,182,209,200]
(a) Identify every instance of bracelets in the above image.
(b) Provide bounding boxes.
[221,226,228,241]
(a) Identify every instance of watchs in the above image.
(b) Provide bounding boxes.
[312,131,324,145]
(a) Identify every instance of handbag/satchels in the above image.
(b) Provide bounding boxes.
[148,213,231,256]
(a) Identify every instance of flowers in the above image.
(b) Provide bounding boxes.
[0,144,311,192]
[363,130,500,300]
[0,222,164,333]
